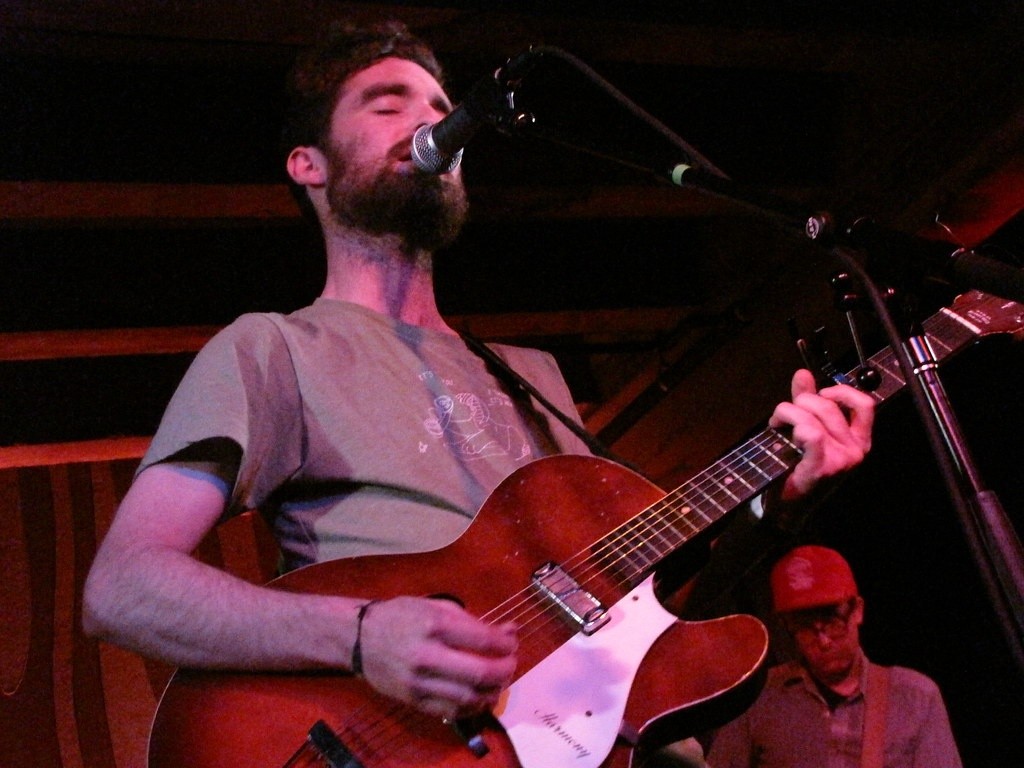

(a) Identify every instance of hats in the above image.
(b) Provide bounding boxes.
[769,545,858,613]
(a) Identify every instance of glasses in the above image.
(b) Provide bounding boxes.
[786,597,857,647]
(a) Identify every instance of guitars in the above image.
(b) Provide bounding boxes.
[140,278,1024,768]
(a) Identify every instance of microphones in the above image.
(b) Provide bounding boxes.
[410,45,546,176]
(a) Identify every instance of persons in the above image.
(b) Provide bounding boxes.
[81,19,875,724]
[694,546,965,768]
